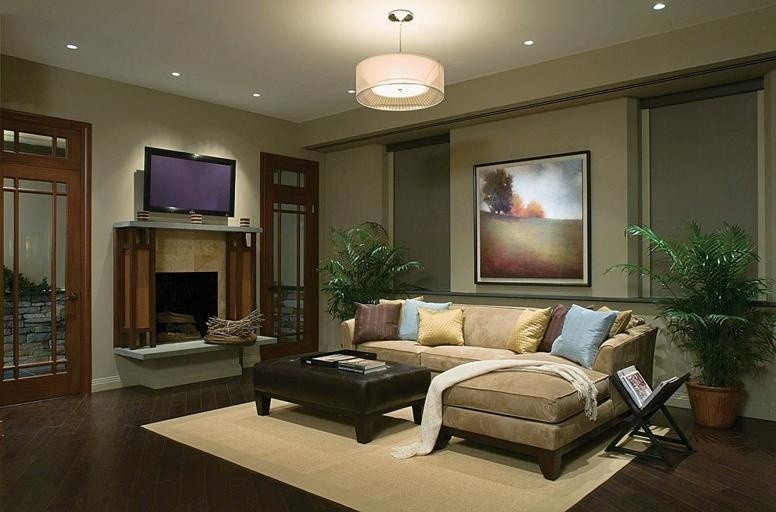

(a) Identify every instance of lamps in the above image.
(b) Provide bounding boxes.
[352,8,446,114]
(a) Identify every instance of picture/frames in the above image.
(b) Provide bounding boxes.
[472,150,594,287]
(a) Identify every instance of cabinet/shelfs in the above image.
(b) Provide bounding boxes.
[113,218,265,350]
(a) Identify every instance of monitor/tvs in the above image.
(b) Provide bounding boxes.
[143,145,236,217]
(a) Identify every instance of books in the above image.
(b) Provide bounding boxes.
[338,365,390,374]
[338,358,385,370]
[616,364,680,411]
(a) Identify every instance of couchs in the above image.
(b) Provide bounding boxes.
[340,304,659,481]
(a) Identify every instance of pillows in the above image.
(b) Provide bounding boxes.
[350,295,646,372]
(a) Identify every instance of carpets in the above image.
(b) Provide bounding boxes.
[138,395,672,512]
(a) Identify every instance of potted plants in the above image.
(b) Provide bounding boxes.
[602,218,775,428]
